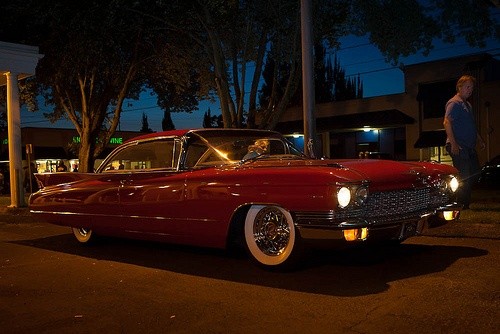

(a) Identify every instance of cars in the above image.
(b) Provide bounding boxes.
[480,154,500,182]
[26,127,466,274]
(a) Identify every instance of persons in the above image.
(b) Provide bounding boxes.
[444,75,486,210]
